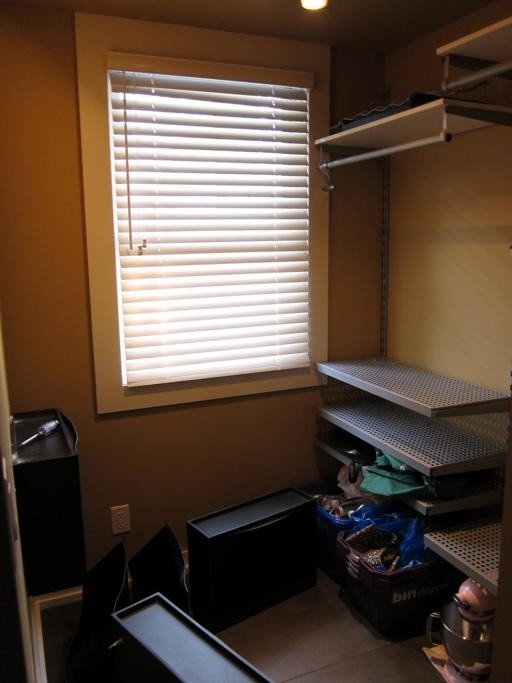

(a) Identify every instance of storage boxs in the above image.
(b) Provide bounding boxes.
[305,479,385,583]
[337,518,466,644]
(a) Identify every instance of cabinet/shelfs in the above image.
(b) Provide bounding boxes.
[317,359,512,518]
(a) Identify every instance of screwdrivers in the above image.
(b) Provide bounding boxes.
[17,419,59,450]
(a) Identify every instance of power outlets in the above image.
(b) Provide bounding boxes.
[110,504,131,535]
